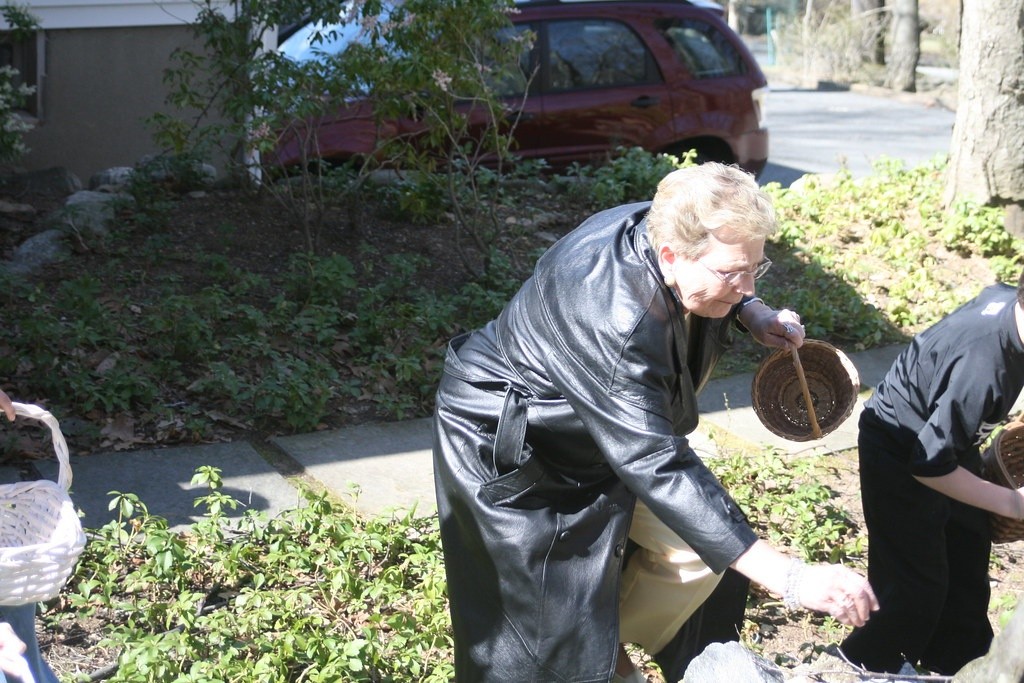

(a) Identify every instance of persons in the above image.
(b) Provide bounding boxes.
[0,391,61,683]
[432,164,881,683]
[835,269,1023,683]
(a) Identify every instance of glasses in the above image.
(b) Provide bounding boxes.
[696,256,774,287]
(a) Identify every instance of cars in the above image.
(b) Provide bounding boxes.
[243,1,770,188]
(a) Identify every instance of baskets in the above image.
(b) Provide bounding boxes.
[981,420,1024,545]
[0,401,88,608]
[751,339,858,443]
